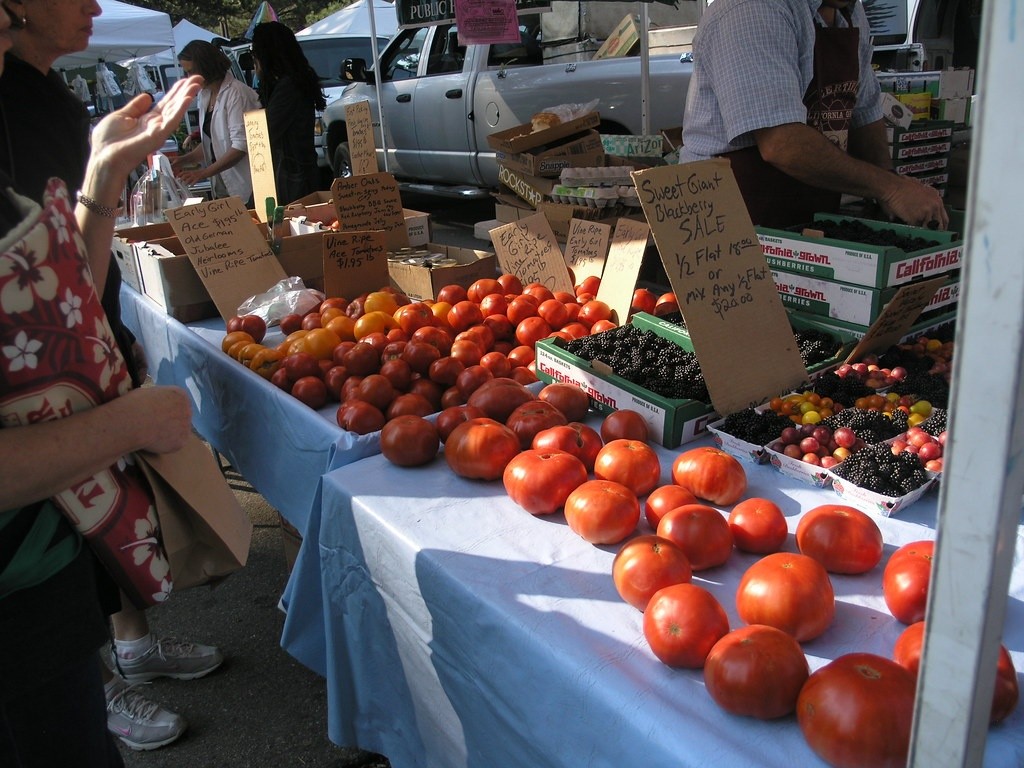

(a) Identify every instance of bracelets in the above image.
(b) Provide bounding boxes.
[76,190,125,217]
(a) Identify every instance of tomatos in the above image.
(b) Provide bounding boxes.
[332,221,340,228]
[223,274,676,436]
[381,378,1018,768]
[835,336,954,387]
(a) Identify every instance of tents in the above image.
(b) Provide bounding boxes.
[50,0,541,135]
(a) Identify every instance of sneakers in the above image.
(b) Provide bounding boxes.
[114,636,224,682]
[104,682,185,752]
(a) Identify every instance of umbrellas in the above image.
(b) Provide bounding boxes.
[245,1,280,41]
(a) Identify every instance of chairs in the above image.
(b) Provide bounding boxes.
[438,31,467,73]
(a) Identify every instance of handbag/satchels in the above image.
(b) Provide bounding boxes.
[138,432,253,591]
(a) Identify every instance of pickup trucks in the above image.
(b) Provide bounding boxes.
[321,19,694,197]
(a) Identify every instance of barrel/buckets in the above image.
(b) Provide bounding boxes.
[158,132,180,180]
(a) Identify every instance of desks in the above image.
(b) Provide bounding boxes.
[119,281,1024,768]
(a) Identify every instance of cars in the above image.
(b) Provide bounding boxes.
[222,34,393,167]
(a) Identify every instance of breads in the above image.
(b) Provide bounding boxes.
[530,113,560,133]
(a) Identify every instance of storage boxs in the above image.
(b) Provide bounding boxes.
[105,109,671,319]
[876,69,977,198]
[753,211,962,378]
[535,311,725,450]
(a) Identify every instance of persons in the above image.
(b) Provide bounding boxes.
[656,0,950,287]
[171,21,330,209]
[0,0,205,768]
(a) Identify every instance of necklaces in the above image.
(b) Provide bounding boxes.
[211,96,215,111]
